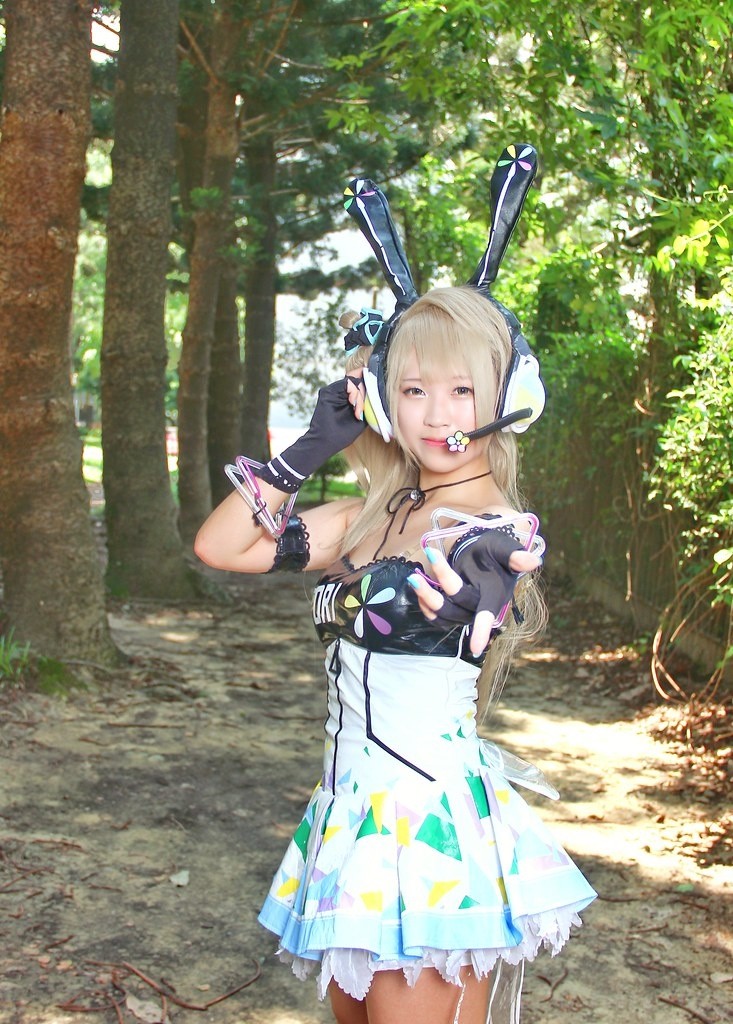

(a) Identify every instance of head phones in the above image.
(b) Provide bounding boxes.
[344,145,544,445]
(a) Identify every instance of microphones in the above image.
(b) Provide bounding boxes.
[446,409,534,453]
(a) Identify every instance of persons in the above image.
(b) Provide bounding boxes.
[194,144,598,1024]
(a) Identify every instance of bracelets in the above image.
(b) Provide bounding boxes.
[419,507,546,628]
[224,456,299,539]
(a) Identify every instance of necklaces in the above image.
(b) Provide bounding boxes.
[372,465,492,561]
